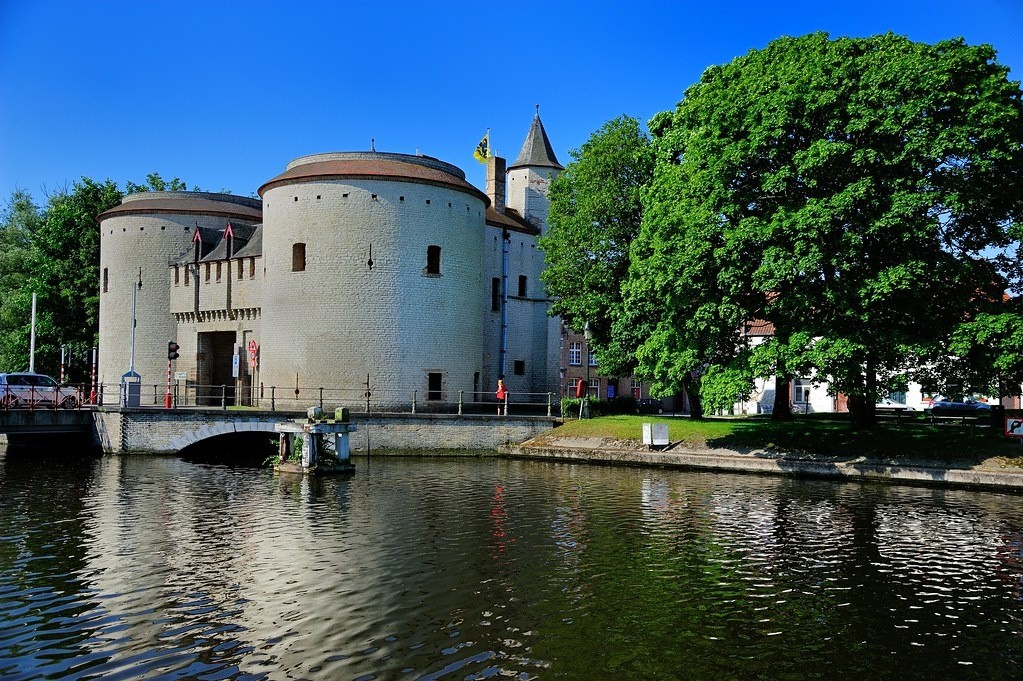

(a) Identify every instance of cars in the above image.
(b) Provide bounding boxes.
[876,397,915,412]
[929,393,991,413]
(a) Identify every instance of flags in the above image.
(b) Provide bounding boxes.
[473,133,491,164]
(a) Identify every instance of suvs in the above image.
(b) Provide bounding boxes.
[0,371,80,410]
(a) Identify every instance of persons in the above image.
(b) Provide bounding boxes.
[495,380,509,415]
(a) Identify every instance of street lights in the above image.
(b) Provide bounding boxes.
[584,320,594,420]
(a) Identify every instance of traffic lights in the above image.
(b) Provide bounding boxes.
[168,342,180,359]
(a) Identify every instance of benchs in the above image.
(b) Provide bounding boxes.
[875,410,990,428]
